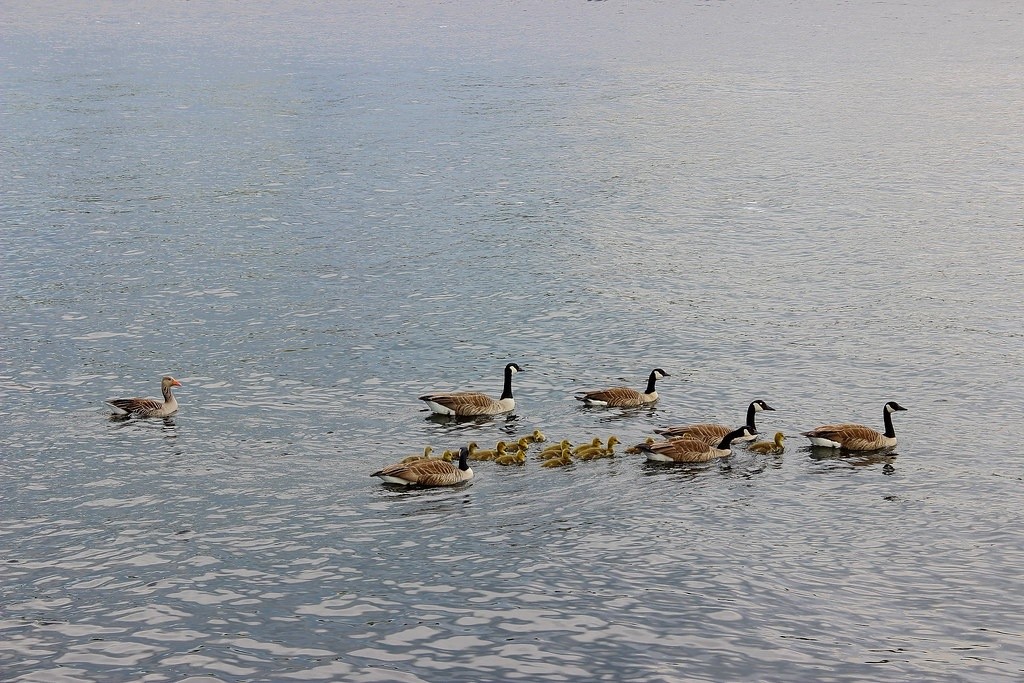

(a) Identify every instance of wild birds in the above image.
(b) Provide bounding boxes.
[106,376,181,417]
[653,400,776,446]
[417,363,525,416]
[542,450,574,467]
[545,440,574,450]
[625,438,654,454]
[495,450,527,464]
[573,368,671,407]
[635,426,762,463]
[371,447,474,486]
[523,431,546,444]
[572,437,622,459]
[505,437,528,452]
[403,447,434,463]
[749,433,788,453]
[452,442,507,461]
[799,401,908,451]
[539,449,572,460]
[432,450,452,463]
